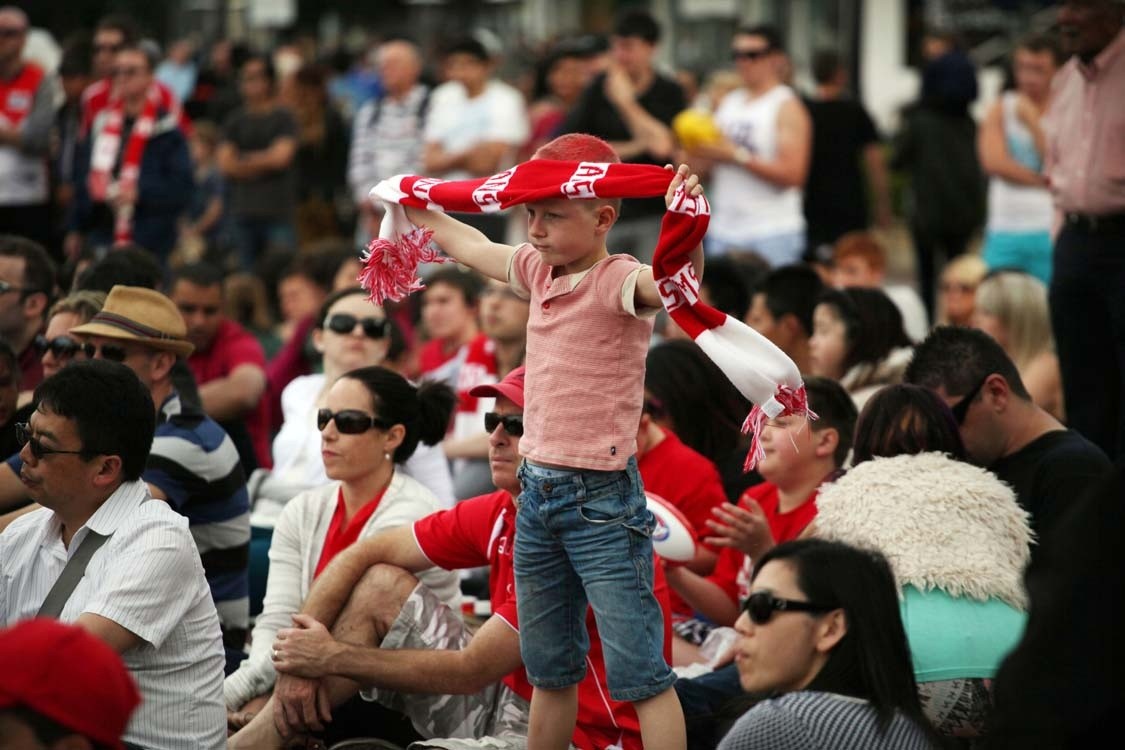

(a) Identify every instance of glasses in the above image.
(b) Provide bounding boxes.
[82,344,127,361]
[739,590,809,626]
[729,48,773,63]
[324,313,392,339]
[14,423,90,460]
[317,408,383,434]
[35,335,86,357]
[484,411,524,437]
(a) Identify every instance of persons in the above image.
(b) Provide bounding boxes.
[0,0,1125,750]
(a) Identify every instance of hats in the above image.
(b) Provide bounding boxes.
[469,366,525,410]
[47,290,106,319]
[69,285,194,357]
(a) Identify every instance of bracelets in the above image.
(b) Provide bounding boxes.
[732,144,749,166]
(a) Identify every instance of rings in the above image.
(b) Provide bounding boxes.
[274,651,278,659]
